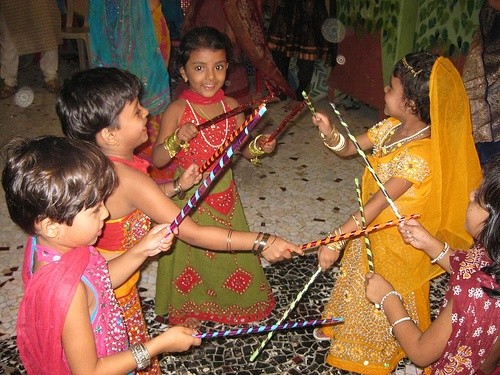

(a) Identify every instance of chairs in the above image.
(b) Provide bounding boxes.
[61,0,92,71]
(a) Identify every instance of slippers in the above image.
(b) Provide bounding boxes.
[313,329,334,340]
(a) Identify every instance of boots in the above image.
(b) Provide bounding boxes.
[270,50,291,101]
[296,58,315,100]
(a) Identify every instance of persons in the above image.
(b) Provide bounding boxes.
[148,25,286,326]
[51,66,307,347]
[363,152,500,375]
[310,48,467,374]
[0,134,204,375]
[0,0,334,130]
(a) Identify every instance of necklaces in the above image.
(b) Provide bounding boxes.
[195,102,223,130]
[186,97,229,148]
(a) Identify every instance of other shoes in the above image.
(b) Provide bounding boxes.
[45,79,61,92]
[1,83,18,98]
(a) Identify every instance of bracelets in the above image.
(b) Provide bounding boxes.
[226,226,278,256]
[324,225,348,253]
[380,291,402,313]
[130,341,151,371]
[318,125,347,151]
[163,176,186,201]
[162,126,191,158]
[430,241,450,265]
[389,317,417,338]
[249,133,268,167]
